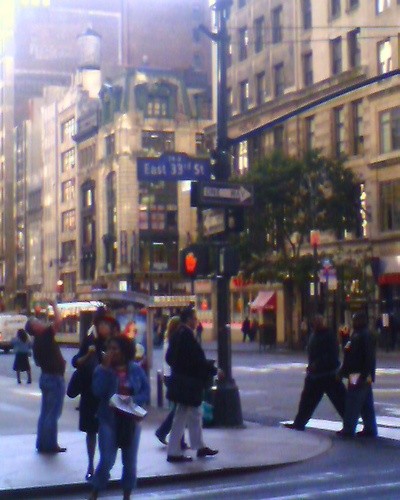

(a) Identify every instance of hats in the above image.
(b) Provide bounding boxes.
[93,306,117,326]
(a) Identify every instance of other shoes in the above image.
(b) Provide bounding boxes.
[38,447,66,453]
[196,447,218,457]
[86,468,94,480]
[181,443,187,449]
[168,455,192,462]
[357,430,376,439]
[155,432,167,444]
[284,424,303,430]
[335,428,355,438]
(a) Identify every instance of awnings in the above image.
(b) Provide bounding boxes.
[250,289,277,311]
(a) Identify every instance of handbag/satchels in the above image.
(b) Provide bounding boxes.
[67,368,90,398]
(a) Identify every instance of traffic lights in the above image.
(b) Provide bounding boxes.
[179,244,204,279]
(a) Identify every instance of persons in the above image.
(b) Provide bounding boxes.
[238,316,259,343]
[335,311,378,437]
[11,299,224,500]
[282,314,357,430]
